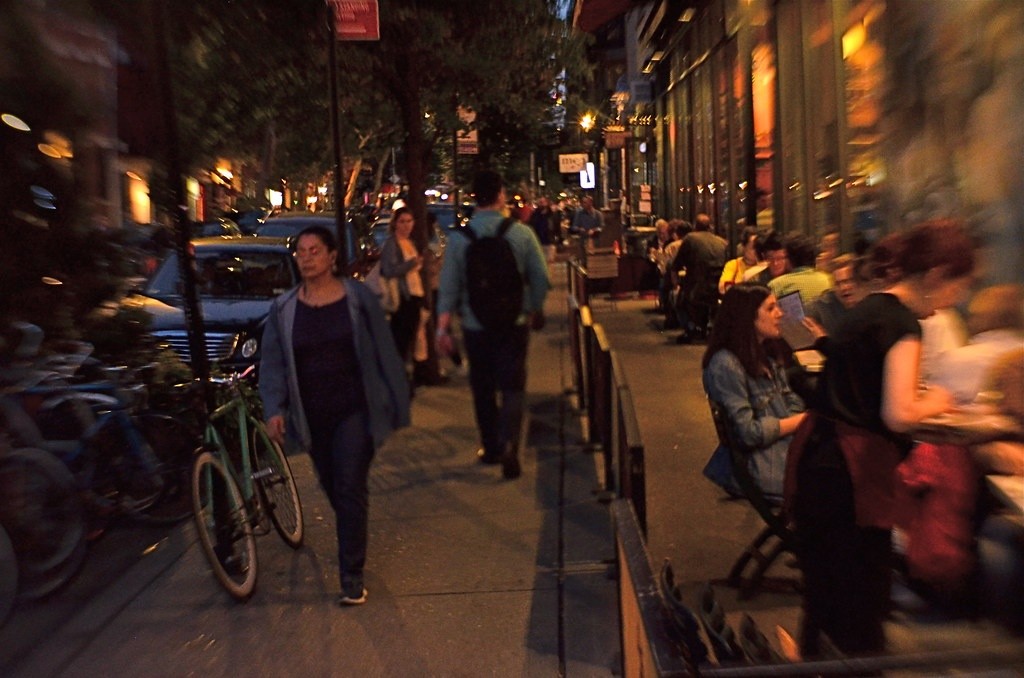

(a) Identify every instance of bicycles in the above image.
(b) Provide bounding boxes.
[0,363,303,618]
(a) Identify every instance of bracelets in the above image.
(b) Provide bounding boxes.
[436,328,451,335]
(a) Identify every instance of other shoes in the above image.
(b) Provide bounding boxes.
[477,448,498,464]
[500,443,520,481]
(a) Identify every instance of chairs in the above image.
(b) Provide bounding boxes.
[706,398,807,593]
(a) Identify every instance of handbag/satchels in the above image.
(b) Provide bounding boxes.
[362,259,399,314]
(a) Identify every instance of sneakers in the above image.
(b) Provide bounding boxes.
[338,585,368,604]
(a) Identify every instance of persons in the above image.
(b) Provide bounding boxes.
[435,177,550,479]
[703,282,810,500]
[106,198,258,276]
[792,221,960,678]
[655,211,1023,623]
[259,225,409,604]
[381,208,425,402]
[513,193,604,268]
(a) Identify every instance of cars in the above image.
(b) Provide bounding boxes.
[428,202,459,234]
[190,198,338,249]
[119,234,309,383]
[358,202,391,248]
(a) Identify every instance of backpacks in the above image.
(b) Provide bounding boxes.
[454,218,523,331]
